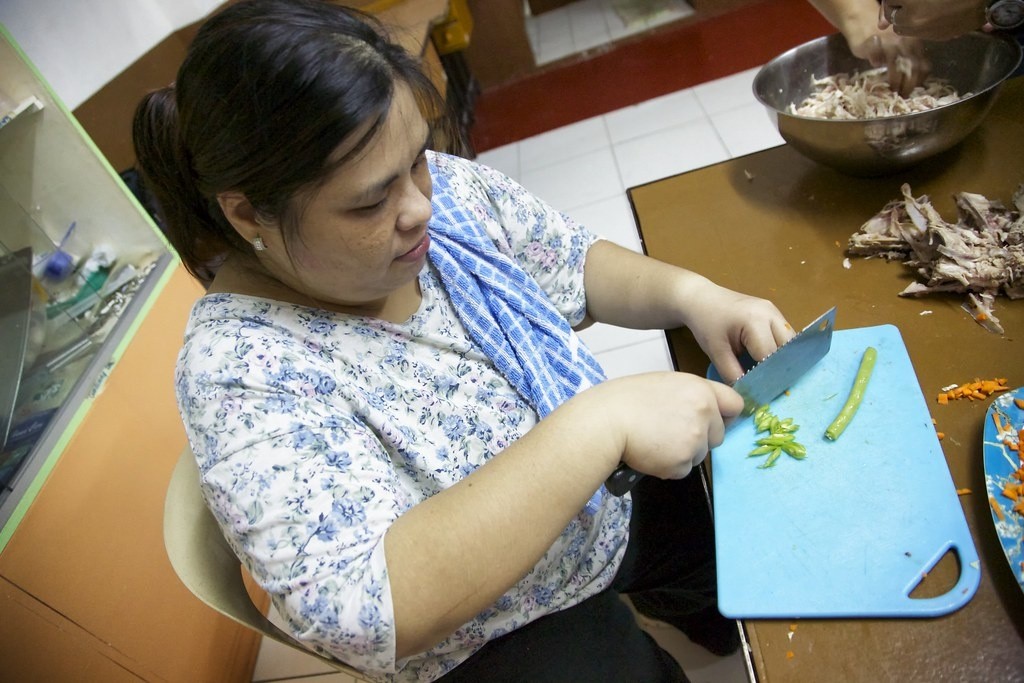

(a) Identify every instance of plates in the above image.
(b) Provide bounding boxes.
[983,385,1024,595]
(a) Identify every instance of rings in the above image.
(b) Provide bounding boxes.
[890,8,899,26]
[896,26,903,35]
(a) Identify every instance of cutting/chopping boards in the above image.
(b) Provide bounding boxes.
[704,323,983,620]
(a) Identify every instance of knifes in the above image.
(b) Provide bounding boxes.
[602,305,841,497]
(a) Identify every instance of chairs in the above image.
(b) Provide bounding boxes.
[163,443,666,683]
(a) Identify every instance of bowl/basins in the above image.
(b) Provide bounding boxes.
[751,28,1024,171]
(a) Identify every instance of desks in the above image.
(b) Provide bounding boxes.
[365,0,482,125]
[388,19,477,160]
[625,74,1024,683]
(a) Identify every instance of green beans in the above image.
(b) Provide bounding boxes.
[824,348,876,440]
[745,405,805,467]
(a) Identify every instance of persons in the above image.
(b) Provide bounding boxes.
[131,1,797,681]
[873,0,1024,44]
[805,0,933,97]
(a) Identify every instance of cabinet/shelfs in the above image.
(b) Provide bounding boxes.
[0,25,262,683]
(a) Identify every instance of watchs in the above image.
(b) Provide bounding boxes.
[981,0,1024,31]
